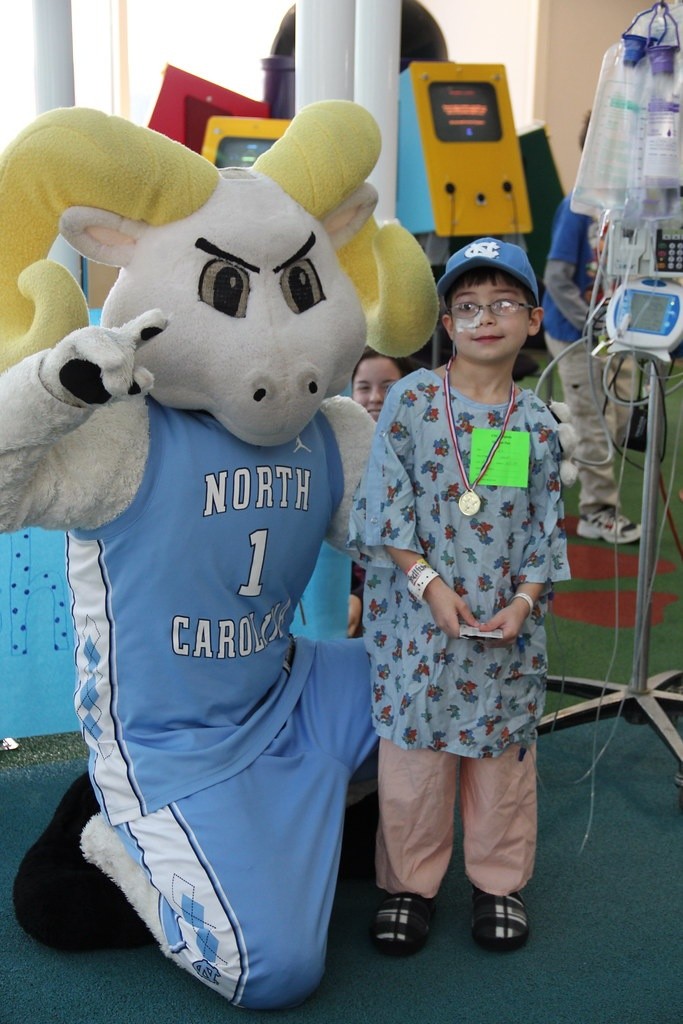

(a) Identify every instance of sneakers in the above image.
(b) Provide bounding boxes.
[576,505,641,543]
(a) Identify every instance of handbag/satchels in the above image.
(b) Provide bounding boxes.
[621,405,647,452]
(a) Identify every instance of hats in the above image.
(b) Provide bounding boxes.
[436,238,540,308]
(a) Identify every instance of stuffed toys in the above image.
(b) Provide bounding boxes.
[1,99,441,1008]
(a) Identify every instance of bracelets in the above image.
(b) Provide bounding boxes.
[404,558,439,604]
[510,593,533,621]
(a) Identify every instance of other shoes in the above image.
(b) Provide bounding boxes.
[371,889,436,957]
[471,882,530,951]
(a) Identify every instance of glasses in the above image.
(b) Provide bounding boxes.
[449,298,534,319]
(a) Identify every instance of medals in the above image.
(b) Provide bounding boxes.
[458,490,481,516]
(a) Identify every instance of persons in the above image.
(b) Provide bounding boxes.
[541,112,644,544]
[347,351,429,637]
[346,239,573,954]
